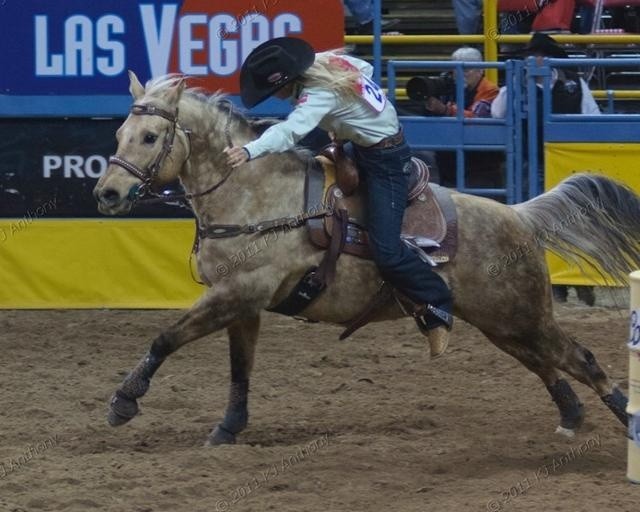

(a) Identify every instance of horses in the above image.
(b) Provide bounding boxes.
[88,64,640,449]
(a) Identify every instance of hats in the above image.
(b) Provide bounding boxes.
[522,33,568,59]
[240,38,315,109]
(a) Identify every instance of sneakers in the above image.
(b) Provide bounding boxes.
[554,286,595,306]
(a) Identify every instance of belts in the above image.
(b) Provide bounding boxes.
[358,122,403,148]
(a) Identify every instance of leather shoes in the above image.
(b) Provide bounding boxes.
[428,327,448,358]
[363,18,399,34]
